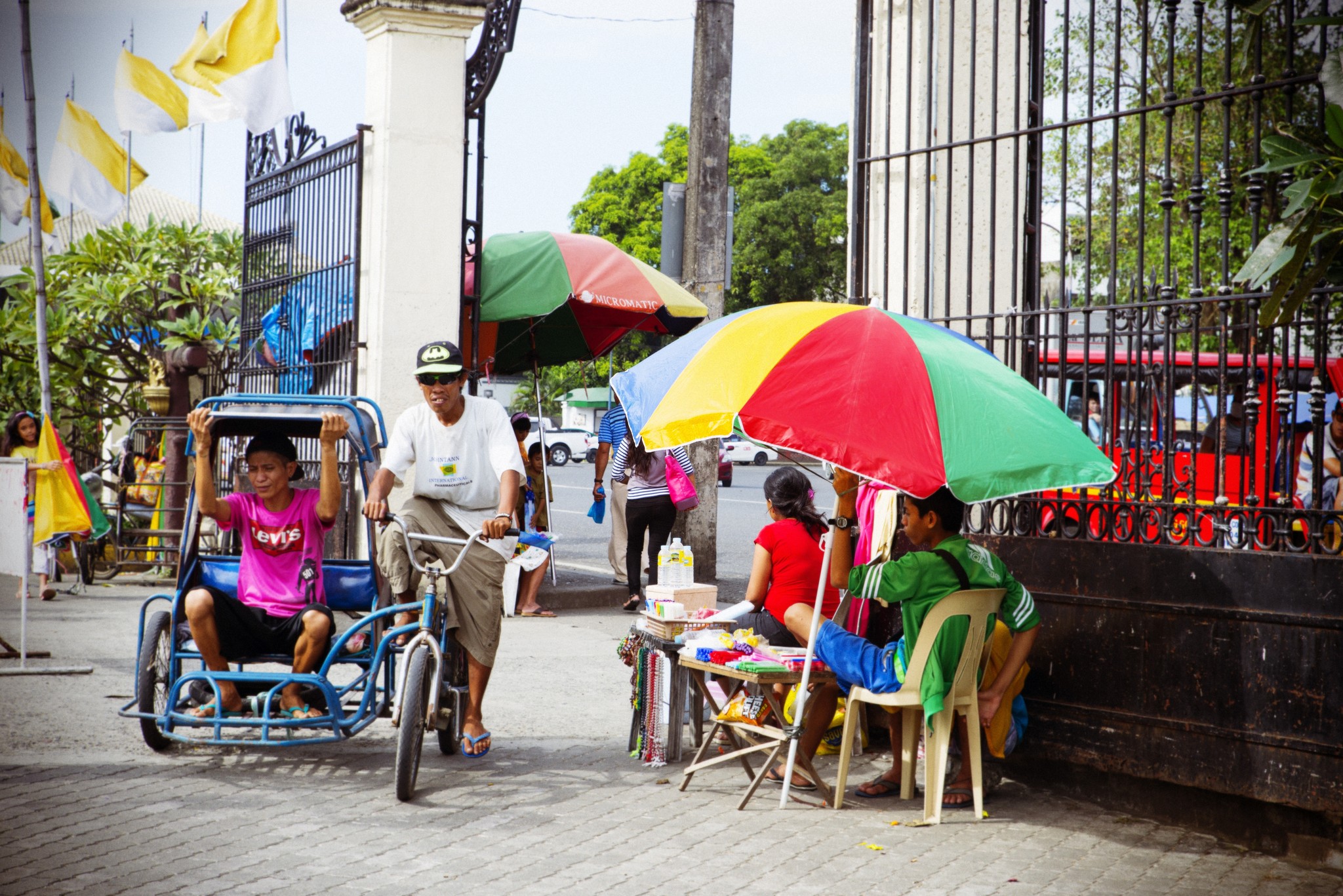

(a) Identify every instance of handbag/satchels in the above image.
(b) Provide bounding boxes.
[664,454,700,512]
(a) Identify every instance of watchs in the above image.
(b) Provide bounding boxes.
[827,516,855,530]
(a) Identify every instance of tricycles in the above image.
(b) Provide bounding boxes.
[1019,346,1342,555]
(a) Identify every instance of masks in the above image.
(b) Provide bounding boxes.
[1230,402,1243,418]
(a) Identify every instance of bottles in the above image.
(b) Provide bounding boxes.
[658,545,671,589]
[669,538,686,588]
[682,545,694,586]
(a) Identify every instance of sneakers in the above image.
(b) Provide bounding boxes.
[611,578,629,585]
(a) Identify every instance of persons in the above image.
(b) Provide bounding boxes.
[0,410,60,603]
[510,412,553,466]
[522,442,554,573]
[363,340,529,759]
[711,465,847,744]
[1194,388,1343,554]
[851,614,1030,807]
[761,461,1039,788]
[611,405,697,611]
[178,406,352,727]
[512,531,557,620]
[1078,391,1105,425]
[592,380,654,585]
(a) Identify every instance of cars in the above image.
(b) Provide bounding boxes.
[716,440,735,487]
[720,434,779,466]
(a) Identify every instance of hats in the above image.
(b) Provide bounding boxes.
[413,341,467,376]
[245,432,304,481]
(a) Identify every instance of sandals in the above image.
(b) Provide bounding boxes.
[623,594,640,611]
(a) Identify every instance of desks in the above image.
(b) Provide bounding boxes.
[674,652,841,810]
[625,626,749,763]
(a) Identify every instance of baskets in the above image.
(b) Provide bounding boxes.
[639,610,738,642]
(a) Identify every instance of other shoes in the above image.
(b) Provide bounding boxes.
[16,592,31,598]
[39,586,56,601]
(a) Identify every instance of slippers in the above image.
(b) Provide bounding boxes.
[942,785,1000,808]
[514,610,522,614]
[387,626,415,647]
[521,607,557,617]
[855,774,919,798]
[462,732,495,758]
[186,703,243,718]
[765,768,818,791]
[280,703,310,719]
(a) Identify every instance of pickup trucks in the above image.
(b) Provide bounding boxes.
[524,418,588,467]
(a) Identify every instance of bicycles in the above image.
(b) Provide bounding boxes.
[63,449,163,586]
[114,393,521,800]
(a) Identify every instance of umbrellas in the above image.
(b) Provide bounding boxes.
[144,424,175,572]
[453,228,710,588]
[32,410,111,563]
[607,299,1118,814]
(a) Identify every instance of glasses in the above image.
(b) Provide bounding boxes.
[416,373,461,386]
[1334,411,1343,423]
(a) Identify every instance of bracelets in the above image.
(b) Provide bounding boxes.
[594,478,603,483]
[495,513,513,525]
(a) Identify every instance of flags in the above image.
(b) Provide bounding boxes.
[0,0,282,235]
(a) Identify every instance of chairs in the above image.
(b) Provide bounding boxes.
[834,586,1007,826]
